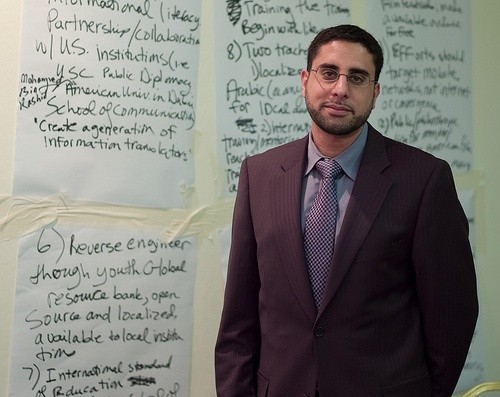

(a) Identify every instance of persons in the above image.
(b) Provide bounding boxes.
[214,25,479,397]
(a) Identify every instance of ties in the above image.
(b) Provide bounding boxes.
[302,157,344,313]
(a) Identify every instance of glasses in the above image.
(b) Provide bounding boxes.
[307,67,378,87]
